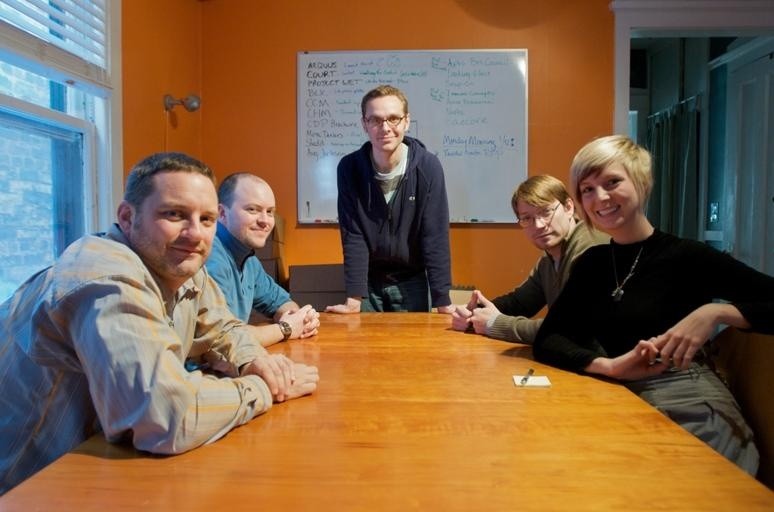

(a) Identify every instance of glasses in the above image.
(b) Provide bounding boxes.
[516,202,561,228]
[364,114,405,126]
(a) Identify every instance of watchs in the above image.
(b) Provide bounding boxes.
[273,321,292,342]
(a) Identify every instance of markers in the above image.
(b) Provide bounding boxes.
[315,219,338,223]
[471,219,494,222]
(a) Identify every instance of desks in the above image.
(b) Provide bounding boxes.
[0,312,774,512]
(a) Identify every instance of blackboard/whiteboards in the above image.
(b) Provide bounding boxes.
[296,48,528,224]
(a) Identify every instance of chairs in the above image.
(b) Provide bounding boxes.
[712,325,774,490]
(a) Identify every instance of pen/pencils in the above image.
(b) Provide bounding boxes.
[521,369,534,384]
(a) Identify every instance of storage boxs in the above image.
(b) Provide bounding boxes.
[267,218,285,244]
[258,259,279,283]
[254,241,279,259]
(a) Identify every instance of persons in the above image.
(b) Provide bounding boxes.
[0,152,320,495]
[533,133,774,478]
[451,173,611,345]
[183,172,320,371]
[324,85,457,313]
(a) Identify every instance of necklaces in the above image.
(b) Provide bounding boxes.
[610,237,643,303]
